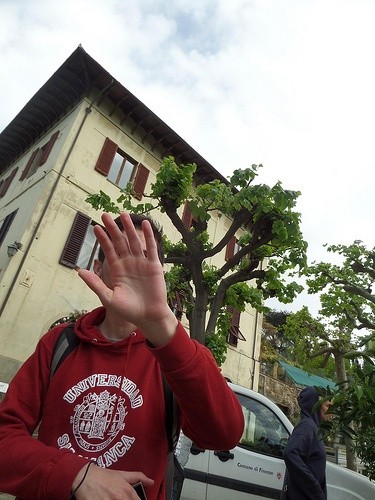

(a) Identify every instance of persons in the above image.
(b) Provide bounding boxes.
[0,212,245,500]
[282,386,332,500]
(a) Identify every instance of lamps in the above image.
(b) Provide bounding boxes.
[7,240,21,257]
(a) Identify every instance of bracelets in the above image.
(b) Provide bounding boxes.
[71,460,98,498]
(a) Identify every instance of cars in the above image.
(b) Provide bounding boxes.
[0,382,375,500]
